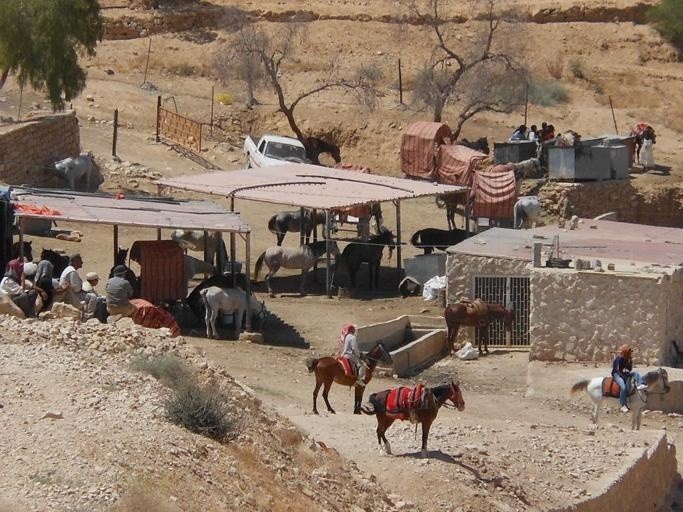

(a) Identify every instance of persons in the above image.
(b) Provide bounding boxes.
[510,122,555,145]
[638,126,655,171]
[0,253,139,321]
[611,344,633,412]
[340,324,367,387]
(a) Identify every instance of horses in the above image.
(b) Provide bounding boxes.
[281,135,341,165]
[410,228,478,255]
[184,254,221,297]
[171,229,229,278]
[436,184,477,231]
[40,248,69,278]
[339,229,395,289]
[306,338,393,415]
[268,207,338,247]
[110,247,137,299]
[513,196,541,229]
[443,302,513,357]
[570,367,671,431]
[13,240,33,262]
[253,240,342,298]
[507,157,542,193]
[199,285,268,340]
[353,379,465,458]
[186,272,252,318]
[629,124,657,164]
[457,136,490,155]
[49,155,98,192]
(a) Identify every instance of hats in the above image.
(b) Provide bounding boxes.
[24,262,37,274]
[114,265,127,275]
[86,272,101,280]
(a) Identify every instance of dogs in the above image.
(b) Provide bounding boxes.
[55,234,81,242]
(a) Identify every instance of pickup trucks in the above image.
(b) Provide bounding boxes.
[243,133,306,170]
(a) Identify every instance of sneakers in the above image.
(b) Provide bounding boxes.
[637,384,648,389]
[620,405,628,412]
[356,380,365,386]
